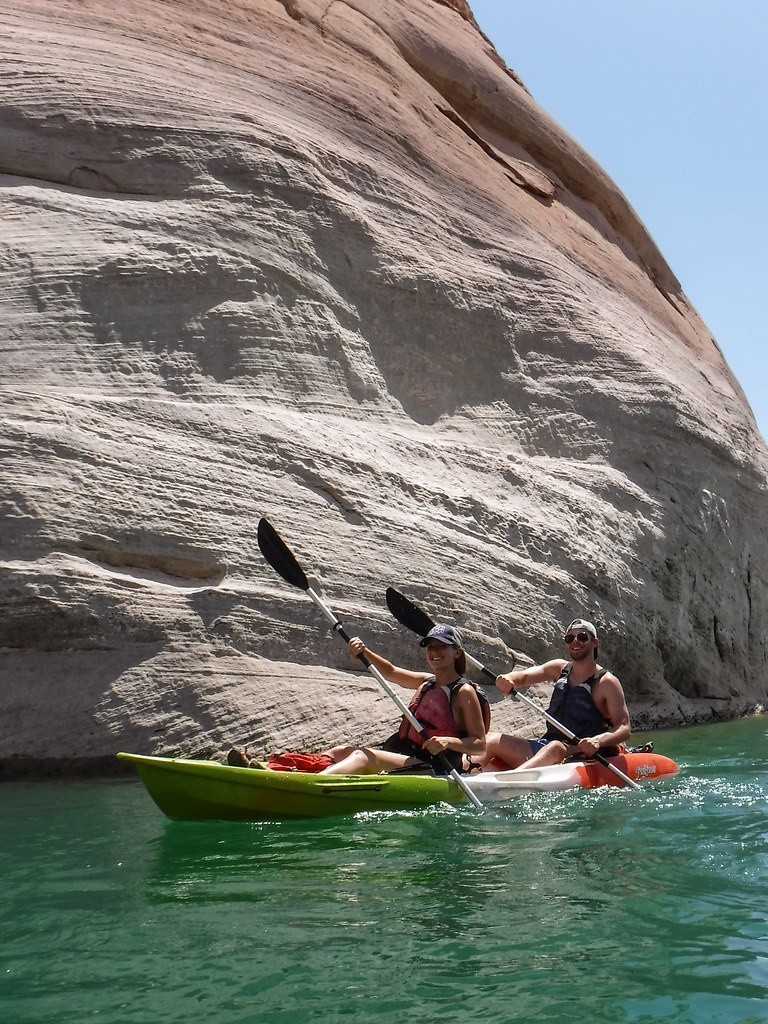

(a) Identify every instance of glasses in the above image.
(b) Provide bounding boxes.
[566,630,593,644]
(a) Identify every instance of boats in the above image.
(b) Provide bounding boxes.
[116,748,680,823]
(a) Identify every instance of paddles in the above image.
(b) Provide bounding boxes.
[257,516,482,807]
[384,585,645,790]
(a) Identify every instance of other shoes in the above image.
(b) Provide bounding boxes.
[248,759,264,770]
[227,750,249,769]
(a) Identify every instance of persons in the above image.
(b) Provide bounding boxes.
[227,624,491,774]
[462,618,631,774]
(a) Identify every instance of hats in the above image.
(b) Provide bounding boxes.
[565,618,598,660]
[420,625,467,676]
